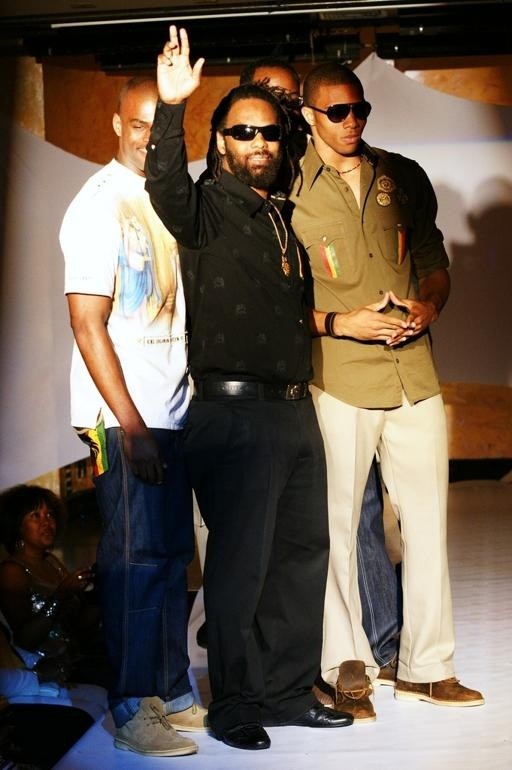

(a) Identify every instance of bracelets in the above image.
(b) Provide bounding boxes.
[324,309,340,340]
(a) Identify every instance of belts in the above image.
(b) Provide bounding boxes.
[204,380,311,399]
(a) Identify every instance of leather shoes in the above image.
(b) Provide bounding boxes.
[292,702,354,727]
[219,722,271,751]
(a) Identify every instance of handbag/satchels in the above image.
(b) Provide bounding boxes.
[60,562,105,649]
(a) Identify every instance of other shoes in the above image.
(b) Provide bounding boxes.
[311,686,332,704]
[377,661,399,686]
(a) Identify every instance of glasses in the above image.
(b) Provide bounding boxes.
[222,123,285,142]
[308,102,373,123]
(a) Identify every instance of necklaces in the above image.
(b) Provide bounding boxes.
[261,196,292,277]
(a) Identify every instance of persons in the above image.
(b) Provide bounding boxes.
[57,68,209,759]
[288,61,486,727]
[142,23,355,751]
[1,484,97,651]
[236,62,400,684]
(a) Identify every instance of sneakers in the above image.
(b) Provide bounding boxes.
[164,703,214,732]
[334,660,376,720]
[394,678,485,707]
[115,696,198,756]
